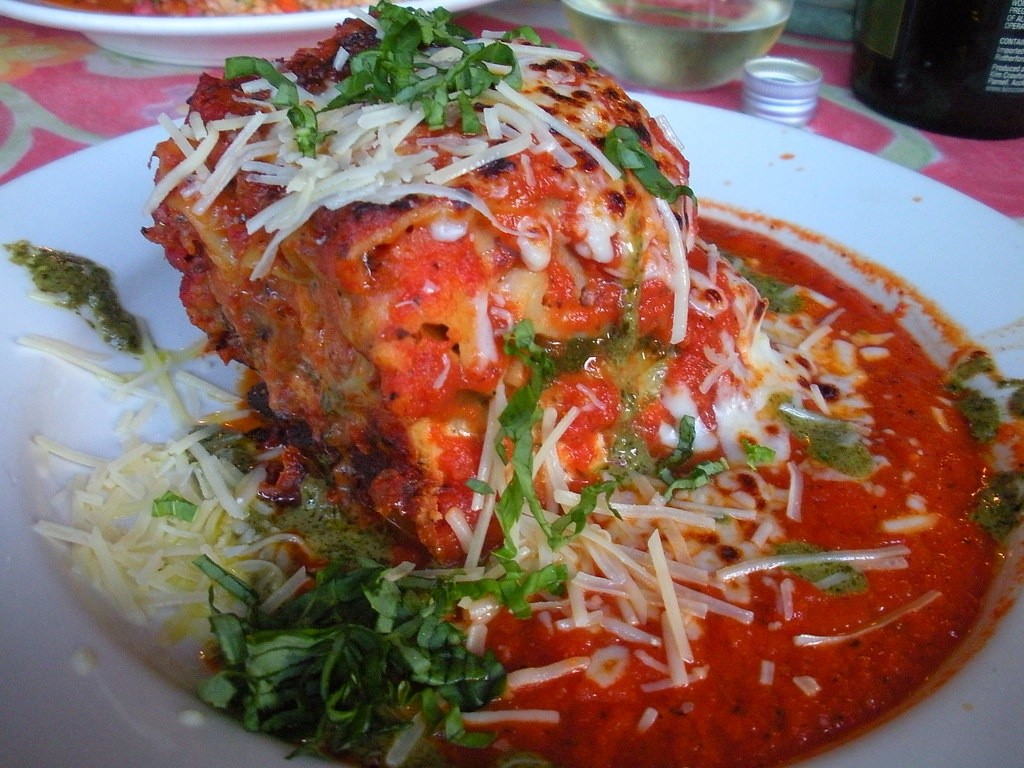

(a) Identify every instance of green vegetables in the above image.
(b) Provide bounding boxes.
[152,0,1024,768]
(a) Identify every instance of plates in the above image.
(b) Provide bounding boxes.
[3,90,1023,768]
[0,0,496,69]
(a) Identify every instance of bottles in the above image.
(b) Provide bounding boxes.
[851,0,1024,140]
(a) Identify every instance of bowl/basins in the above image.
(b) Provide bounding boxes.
[558,0,791,89]
